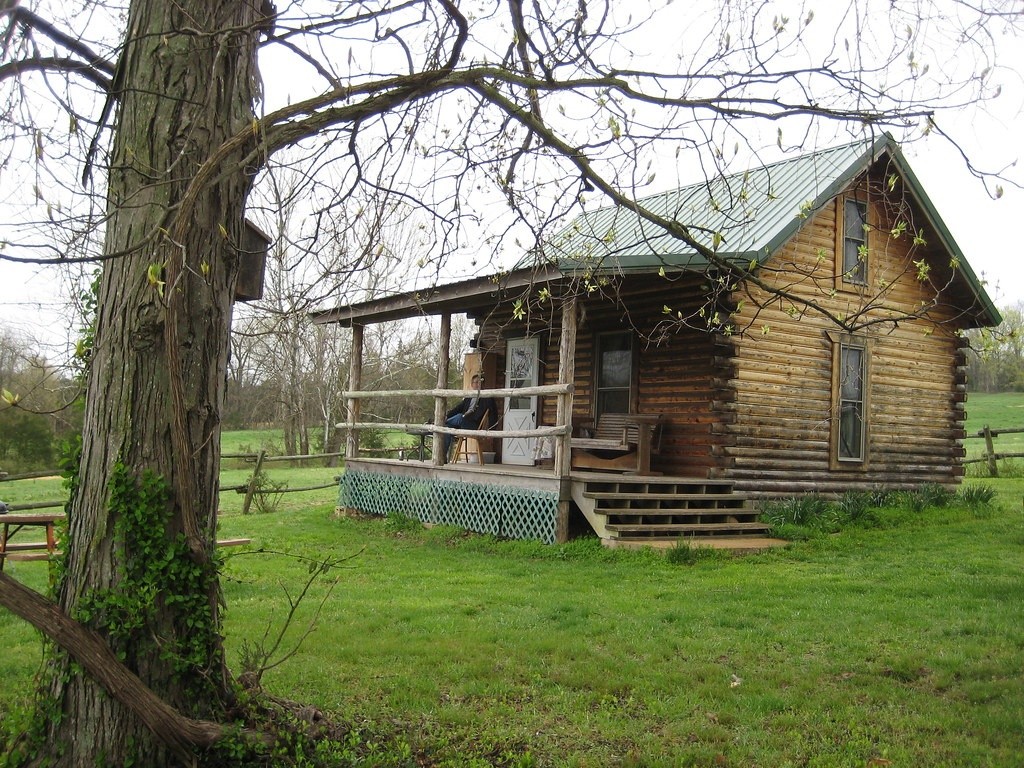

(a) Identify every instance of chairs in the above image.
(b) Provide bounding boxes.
[451,408,490,467]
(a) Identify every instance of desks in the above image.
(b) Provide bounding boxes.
[0,514,66,598]
[406,431,433,462]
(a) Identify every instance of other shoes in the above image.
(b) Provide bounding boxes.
[444,457,447,464]
[428,418,434,424]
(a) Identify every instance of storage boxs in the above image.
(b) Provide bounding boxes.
[469,451,496,464]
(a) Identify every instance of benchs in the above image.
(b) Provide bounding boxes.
[571,413,673,476]
[0,538,251,561]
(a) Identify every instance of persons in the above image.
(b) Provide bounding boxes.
[427,375,498,463]
